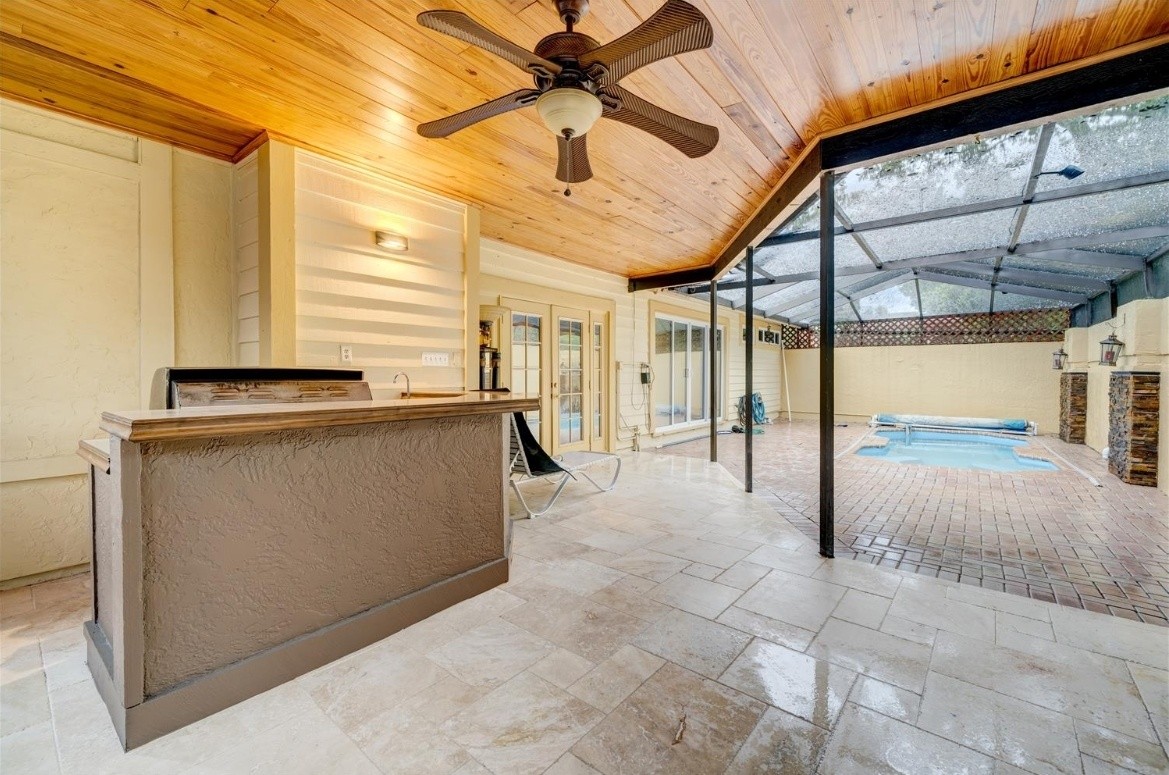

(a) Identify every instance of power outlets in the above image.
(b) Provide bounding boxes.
[340,345,352,361]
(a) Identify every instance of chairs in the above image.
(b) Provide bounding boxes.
[509,412,623,519]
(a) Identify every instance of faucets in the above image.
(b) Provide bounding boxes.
[393,372,410,399]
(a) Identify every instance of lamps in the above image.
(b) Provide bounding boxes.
[376,231,408,251]
[535,61,603,197]
[1098,314,1126,366]
[1051,344,1068,369]
[1033,165,1085,180]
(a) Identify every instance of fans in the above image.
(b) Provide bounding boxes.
[416,0,720,186]
